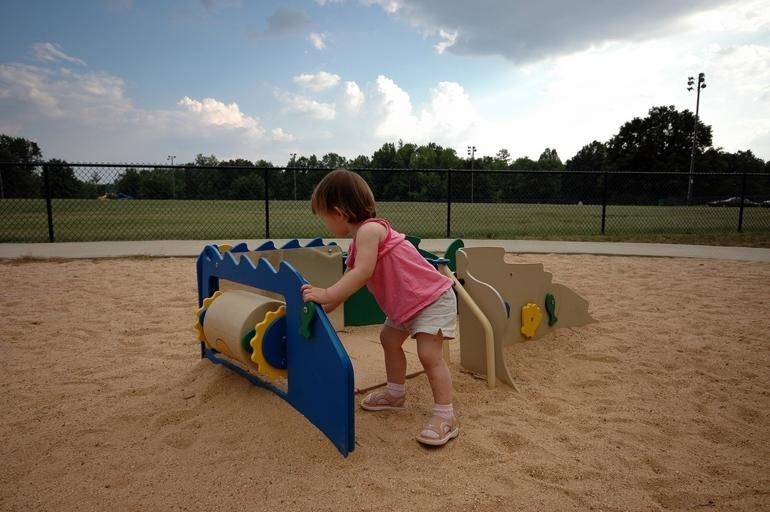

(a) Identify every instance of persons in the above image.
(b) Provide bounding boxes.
[300,168,460,448]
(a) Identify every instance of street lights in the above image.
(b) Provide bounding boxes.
[290,153,297,200]
[468,146,477,204]
[687,72,706,204]
[167,155,176,199]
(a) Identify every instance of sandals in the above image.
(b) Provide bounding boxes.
[360,387,409,411]
[415,414,458,445]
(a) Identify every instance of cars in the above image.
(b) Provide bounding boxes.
[709,196,760,207]
